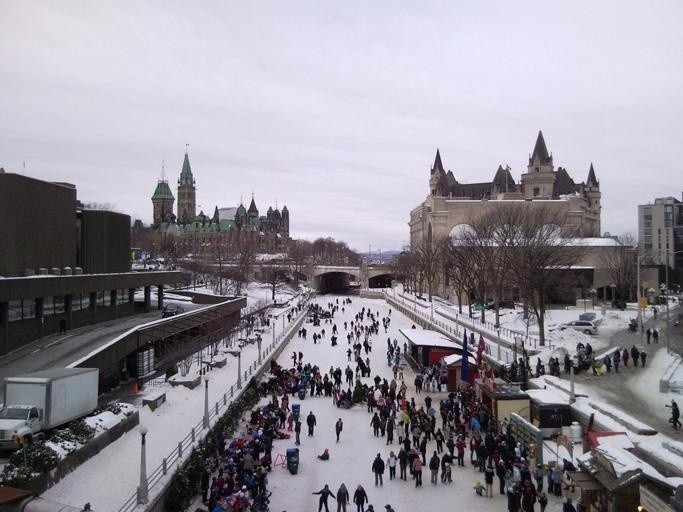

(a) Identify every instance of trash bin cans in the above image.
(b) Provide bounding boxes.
[292,404,300,421]
[297,384,305,400]
[286,448,299,474]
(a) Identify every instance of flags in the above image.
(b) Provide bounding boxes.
[185,144,190,146]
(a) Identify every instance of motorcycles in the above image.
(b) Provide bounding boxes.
[331,389,351,409]
[286,448,299,474]
[249,490,272,512]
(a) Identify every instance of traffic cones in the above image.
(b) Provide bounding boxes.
[132,383,138,393]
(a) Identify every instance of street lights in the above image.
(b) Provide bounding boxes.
[151,242,155,258]
[399,245,416,295]
[206,244,208,260]
[228,224,231,245]
[272,321,275,347]
[647,288,655,305]
[202,375,210,428]
[255,333,263,364]
[335,248,338,265]
[637,248,670,327]
[138,424,149,505]
[610,283,616,299]
[236,349,241,389]
[341,246,344,264]
[589,288,596,309]
[665,250,682,353]
[282,314,285,335]
[376,248,381,265]
[272,233,281,300]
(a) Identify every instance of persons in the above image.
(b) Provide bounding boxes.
[59,315,68,337]
[665,399,682,429]
[646,328,660,344]
[202,350,342,512]
[346,306,392,377]
[342,358,488,487]
[479,427,587,512]
[337,483,395,512]
[246,297,353,347]
[511,345,647,381]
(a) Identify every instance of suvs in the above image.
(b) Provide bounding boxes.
[485,301,515,311]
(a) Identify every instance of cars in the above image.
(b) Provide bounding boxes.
[560,320,597,334]
[160,302,184,318]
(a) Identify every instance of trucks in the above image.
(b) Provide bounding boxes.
[0,367,99,451]
